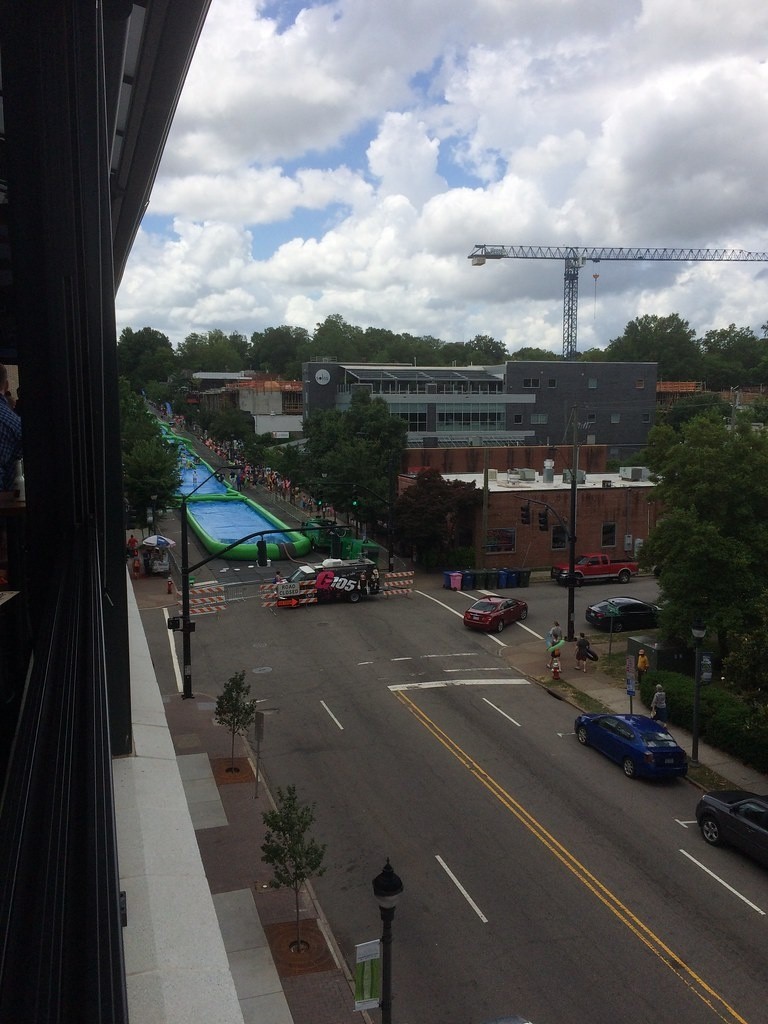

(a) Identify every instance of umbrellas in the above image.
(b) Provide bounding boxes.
[143,534,176,548]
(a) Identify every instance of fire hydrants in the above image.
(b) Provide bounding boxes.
[551,662,562,680]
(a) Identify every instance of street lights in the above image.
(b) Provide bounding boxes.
[371,856,404,1024]
[545,447,574,642]
[149,491,159,536]
[182,464,244,697]
[688,621,708,761]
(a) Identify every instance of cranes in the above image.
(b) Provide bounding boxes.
[466,243,767,362]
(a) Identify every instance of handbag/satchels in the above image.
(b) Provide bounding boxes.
[272,578,277,584]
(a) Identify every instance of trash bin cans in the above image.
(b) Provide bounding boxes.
[442,571,454,590]
[506,569,519,588]
[519,568,531,588]
[486,570,498,590]
[449,573,462,591]
[473,570,487,590]
[440,567,531,591]
[497,571,508,588]
[461,570,475,591]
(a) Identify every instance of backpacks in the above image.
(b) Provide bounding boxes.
[134,560,139,567]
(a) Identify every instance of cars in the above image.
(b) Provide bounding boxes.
[464,595,528,634]
[574,712,689,780]
[585,597,663,632]
[696,789,768,870]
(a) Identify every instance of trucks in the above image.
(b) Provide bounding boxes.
[299,516,380,564]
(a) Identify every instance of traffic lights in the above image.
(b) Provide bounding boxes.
[317,488,324,506]
[538,510,549,531]
[127,509,137,530]
[520,505,530,525]
[351,489,360,508]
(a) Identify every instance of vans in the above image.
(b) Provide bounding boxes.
[276,556,380,604]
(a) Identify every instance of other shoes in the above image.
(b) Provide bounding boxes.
[546,664,551,668]
[574,666,580,669]
[663,724,667,729]
[582,669,586,673]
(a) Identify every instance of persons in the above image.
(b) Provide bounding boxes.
[546,621,562,672]
[573,632,590,672]
[638,649,649,683]
[274,571,281,582]
[0,363,22,492]
[301,495,334,517]
[128,534,138,547]
[652,685,666,725]
[209,442,290,495]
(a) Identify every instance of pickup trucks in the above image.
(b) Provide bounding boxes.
[552,552,640,585]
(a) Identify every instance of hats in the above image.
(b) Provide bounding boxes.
[638,649,645,654]
